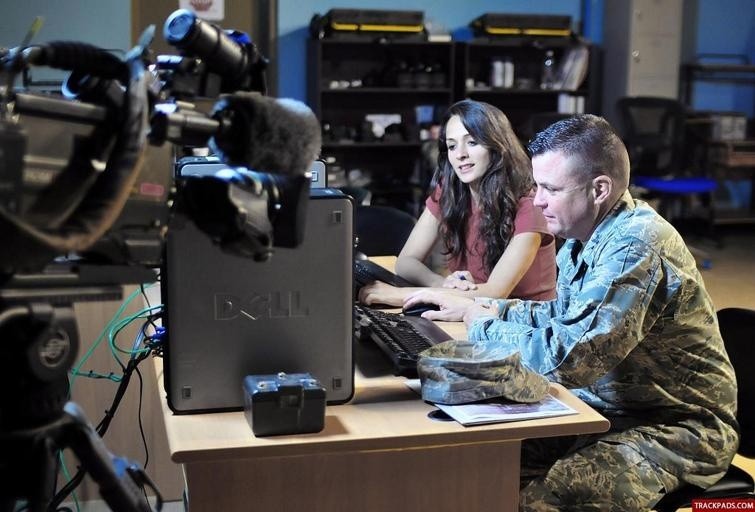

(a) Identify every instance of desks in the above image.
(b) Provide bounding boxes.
[140,255,611,510]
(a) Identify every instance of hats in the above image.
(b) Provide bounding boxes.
[417,339,550,406]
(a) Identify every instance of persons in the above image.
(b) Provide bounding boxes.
[359,98,556,308]
[403,111,738,512]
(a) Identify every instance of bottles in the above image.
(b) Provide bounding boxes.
[542,51,557,90]
[492,56,504,88]
[504,58,514,88]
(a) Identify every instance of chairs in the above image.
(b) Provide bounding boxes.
[652,306,755,512]
[615,94,718,272]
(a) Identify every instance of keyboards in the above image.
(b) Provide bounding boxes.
[353,260,421,300]
[352,305,455,379]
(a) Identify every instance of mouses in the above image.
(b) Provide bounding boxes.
[403,302,441,316]
[354,251,369,260]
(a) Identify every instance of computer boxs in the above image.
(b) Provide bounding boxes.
[162,186,355,412]
[174,155,328,188]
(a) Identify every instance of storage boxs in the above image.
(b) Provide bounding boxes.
[323,6,426,41]
[469,12,574,36]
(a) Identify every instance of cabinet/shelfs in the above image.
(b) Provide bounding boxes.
[307,39,456,219]
[677,53,755,226]
[462,37,607,142]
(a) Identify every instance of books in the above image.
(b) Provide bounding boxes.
[406,378,579,426]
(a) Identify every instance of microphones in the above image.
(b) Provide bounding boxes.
[151,88,323,175]
[164,8,270,85]
